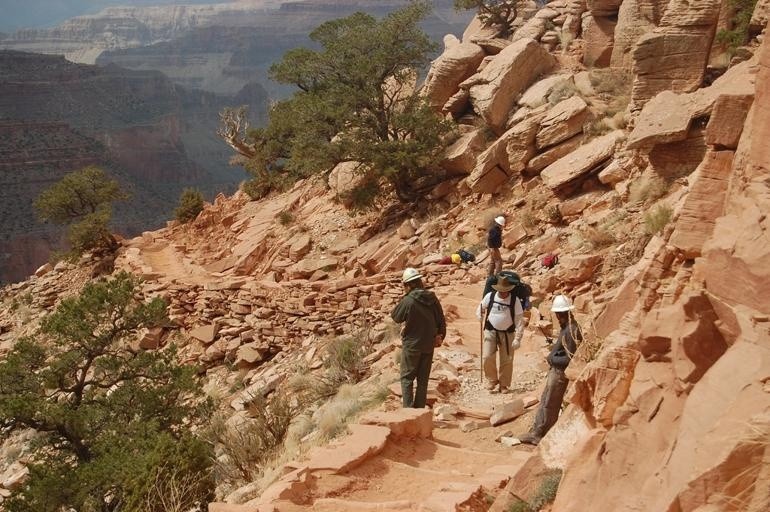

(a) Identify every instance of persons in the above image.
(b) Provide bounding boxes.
[476,276,524,394]
[389,268,447,408]
[486,216,506,278]
[518,295,583,445]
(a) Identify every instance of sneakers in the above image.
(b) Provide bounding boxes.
[517,433,540,447]
[499,386,509,394]
[486,378,499,391]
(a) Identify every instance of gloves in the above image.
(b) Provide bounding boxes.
[511,337,520,350]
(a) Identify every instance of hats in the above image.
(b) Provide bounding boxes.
[490,275,517,293]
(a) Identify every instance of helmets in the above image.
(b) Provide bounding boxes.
[550,295,574,314]
[401,268,423,283]
[495,216,506,227]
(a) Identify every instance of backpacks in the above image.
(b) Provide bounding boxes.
[456,249,476,262]
[541,254,557,269]
